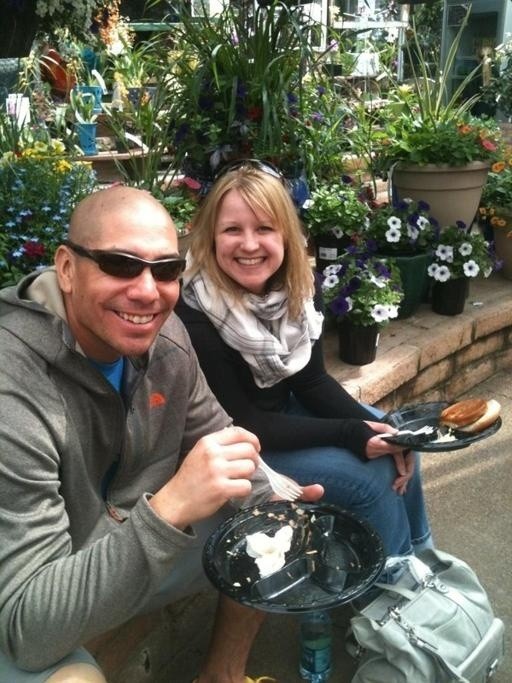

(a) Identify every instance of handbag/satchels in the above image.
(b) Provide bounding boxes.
[350,548,505,683]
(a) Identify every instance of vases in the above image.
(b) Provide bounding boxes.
[341,323,377,365]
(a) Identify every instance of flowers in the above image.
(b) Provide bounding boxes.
[0,0,511,329]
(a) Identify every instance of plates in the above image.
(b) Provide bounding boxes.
[200,499,388,615]
[379,400,503,452]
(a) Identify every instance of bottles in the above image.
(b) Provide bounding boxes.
[298,607,335,683]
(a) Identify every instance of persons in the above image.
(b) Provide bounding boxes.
[1,184,328,682]
[172,161,436,598]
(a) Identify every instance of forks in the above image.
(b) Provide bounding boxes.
[377,424,433,438]
[221,423,303,503]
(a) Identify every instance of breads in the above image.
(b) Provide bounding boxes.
[440,396,502,433]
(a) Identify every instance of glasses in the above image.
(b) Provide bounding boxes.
[213,158,285,186]
[62,238,187,281]
